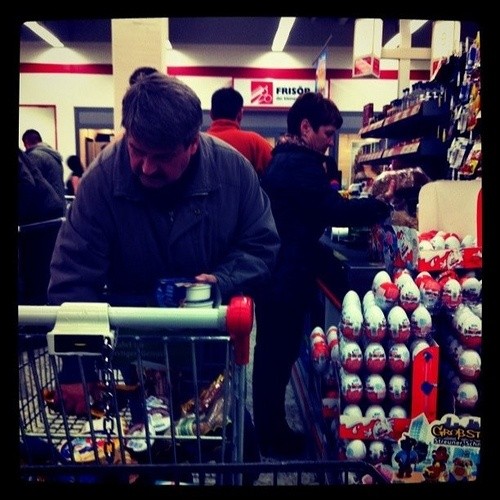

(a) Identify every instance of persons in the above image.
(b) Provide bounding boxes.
[19,148,64,349]
[253,92,418,460]
[22,129,66,209]
[392,187,421,218]
[205,88,274,174]
[66,155,84,201]
[129,67,157,84]
[47,72,280,487]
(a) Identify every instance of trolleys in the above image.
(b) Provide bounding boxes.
[17,295,258,485]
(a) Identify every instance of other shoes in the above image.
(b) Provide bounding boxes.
[255,417,298,443]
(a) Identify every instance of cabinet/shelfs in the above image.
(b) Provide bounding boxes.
[352,101,448,163]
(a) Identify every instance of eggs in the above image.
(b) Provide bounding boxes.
[312,231,482,484]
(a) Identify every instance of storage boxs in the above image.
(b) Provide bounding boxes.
[388,179,483,272]
